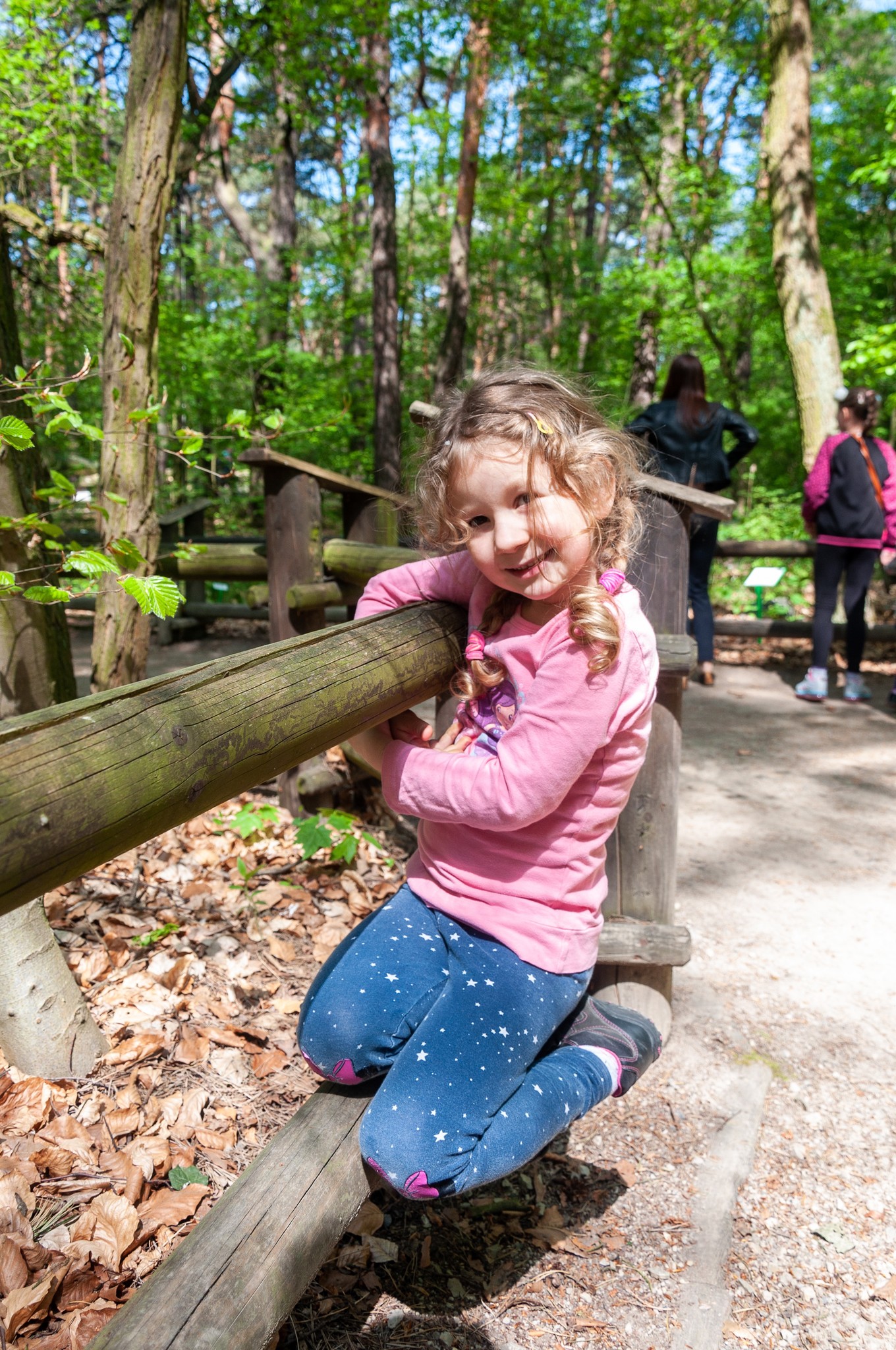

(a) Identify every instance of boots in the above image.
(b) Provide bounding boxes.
[795,668,829,701]
[843,673,873,701]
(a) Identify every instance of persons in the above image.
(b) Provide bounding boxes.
[627,354,759,691]
[795,386,896,702]
[294,363,671,1202]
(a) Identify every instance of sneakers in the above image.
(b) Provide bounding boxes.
[551,998,662,1098]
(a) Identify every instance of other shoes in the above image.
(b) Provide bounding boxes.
[698,671,716,686]
